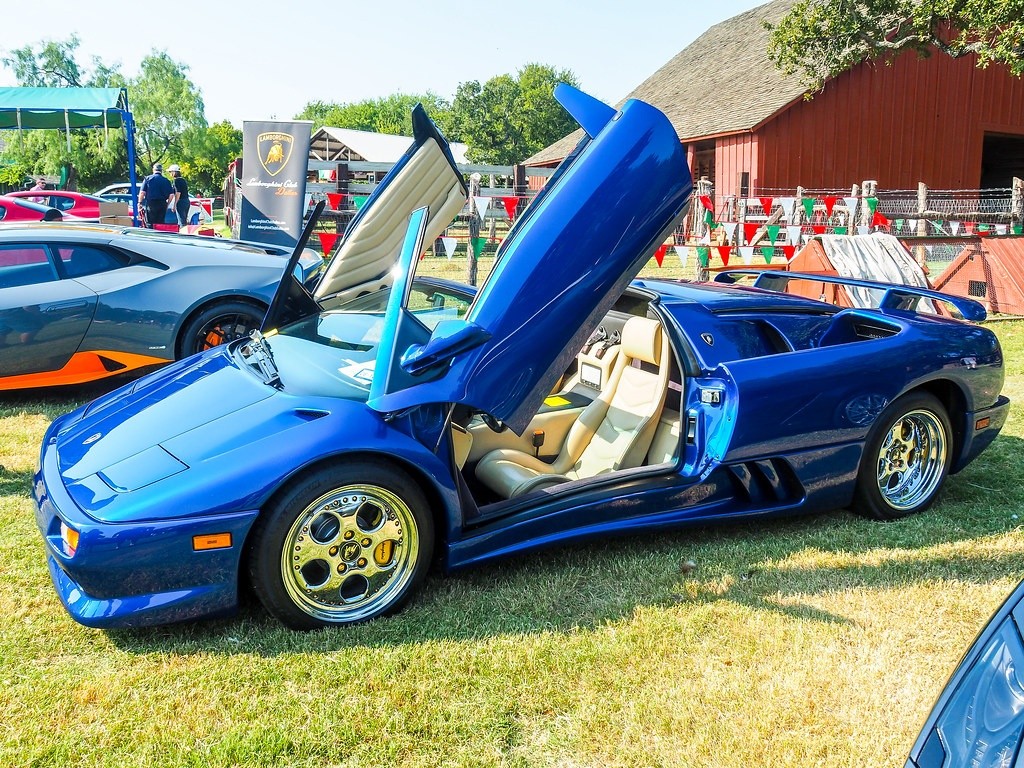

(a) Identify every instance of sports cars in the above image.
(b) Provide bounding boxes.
[0,222,325,402]
[32,84,1013,635]
[0,191,194,267]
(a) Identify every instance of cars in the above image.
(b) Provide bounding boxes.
[92,182,148,206]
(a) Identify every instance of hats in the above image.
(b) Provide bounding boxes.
[40,209,63,221]
[166,164,181,172]
[153,163,163,170]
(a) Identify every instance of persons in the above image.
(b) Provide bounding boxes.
[28,178,47,206]
[40,208,63,221]
[167,165,190,226]
[137,162,174,230]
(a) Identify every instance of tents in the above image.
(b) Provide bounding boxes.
[1,87,125,128]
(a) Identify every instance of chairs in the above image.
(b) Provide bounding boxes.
[68,250,99,276]
[475,316,674,499]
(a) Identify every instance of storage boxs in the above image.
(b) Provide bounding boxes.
[98,201,132,227]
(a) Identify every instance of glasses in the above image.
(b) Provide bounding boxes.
[39,182,47,185]
